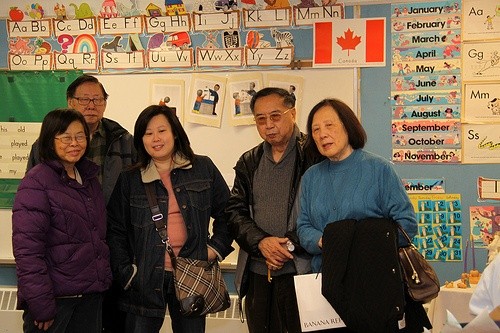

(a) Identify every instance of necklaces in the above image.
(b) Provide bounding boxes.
[74,166,77,180]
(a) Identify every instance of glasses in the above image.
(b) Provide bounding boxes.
[72,96,106,106]
[54,135,88,143]
[253,108,293,124]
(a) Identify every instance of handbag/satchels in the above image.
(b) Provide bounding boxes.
[294,256,348,332]
[394,223,441,304]
[172,257,231,315]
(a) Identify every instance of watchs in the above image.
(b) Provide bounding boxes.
[283,235,296,254]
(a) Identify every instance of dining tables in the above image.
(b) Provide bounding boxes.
[426,275,500,333]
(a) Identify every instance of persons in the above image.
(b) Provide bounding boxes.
[107,103,235,333]
[296,99,440,333]
[19,71,138,211]
[12,107,113,333]
[469,232,500,316]
[224,87,328,333]
